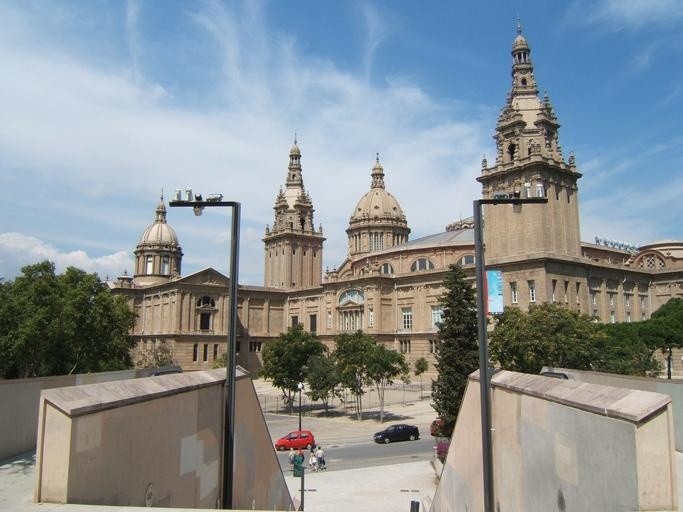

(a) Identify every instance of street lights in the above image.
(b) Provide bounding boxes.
[298,382,302,431]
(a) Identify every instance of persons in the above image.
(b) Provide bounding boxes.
[310,447,325,471]
[289,448,304,477]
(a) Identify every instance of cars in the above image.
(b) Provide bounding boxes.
[275,431,316,451]
[374,424,419,444]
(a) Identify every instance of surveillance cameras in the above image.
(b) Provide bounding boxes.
[534,183,545,197]
[174,189,181,200]
[184,185,194,201]
[522,182,531,199]
